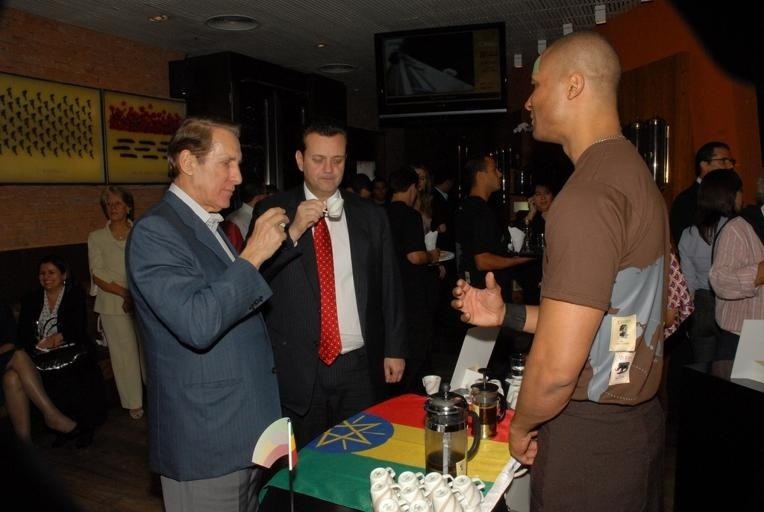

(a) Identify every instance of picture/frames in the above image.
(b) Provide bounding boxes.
[1,70,107,187]
[102,89,189,186]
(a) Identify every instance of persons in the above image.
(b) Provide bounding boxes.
[124,119,557,512]
[664,141,764,512]
[88,186,146,419]
[17,255,109,449]
[452,33,673,512]
[1,297,83,456]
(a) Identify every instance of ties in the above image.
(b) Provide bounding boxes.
[313,209,342,366]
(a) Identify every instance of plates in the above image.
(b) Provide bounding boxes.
[436,249,456,262]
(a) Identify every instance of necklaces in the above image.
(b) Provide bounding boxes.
[112,223,131,240]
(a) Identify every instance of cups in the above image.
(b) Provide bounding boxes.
[471,381,506,439]
[421,382,483,480]
[318,197,344,221]
[503,352,527,412]
[369,466,485,512]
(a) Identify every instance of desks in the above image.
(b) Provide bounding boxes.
[254,392,523,511]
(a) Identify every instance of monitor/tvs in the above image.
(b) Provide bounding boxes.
[372,21,508,119]
